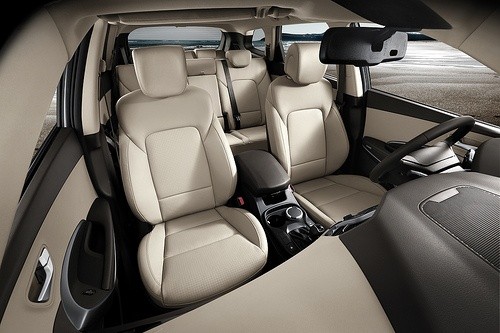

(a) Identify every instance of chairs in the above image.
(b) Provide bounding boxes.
[264,41,387,234]
[182,58,244,155]
[110,45,270,305]
[215,49,272,148]
